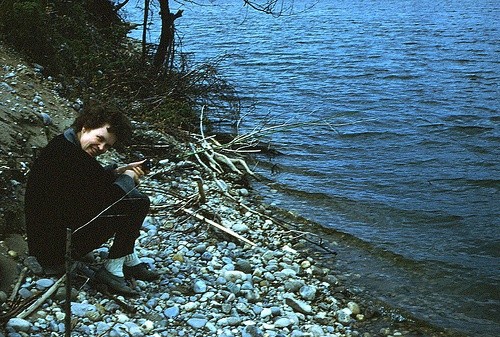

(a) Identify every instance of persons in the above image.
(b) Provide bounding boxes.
[25,107,160,298]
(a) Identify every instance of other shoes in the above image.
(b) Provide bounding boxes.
[123,262,160,281]
[94,267,139,297]
